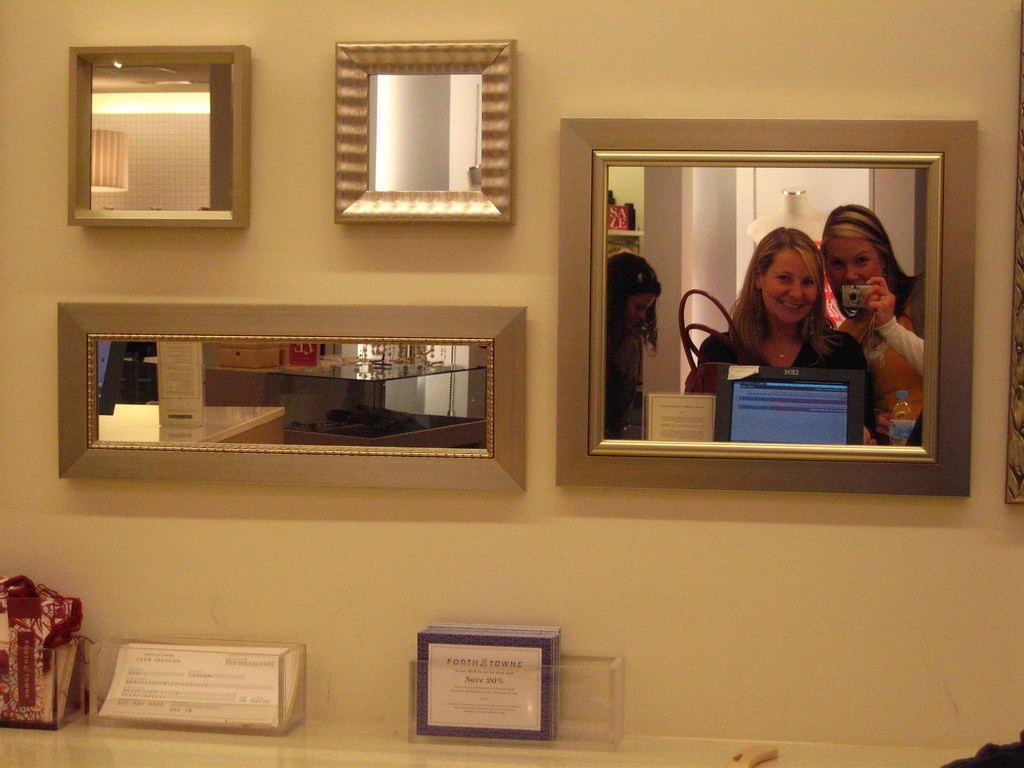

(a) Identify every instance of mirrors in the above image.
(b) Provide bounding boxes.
[56,296,528,496]
[556,118,973,497]
[69,48,248,225]
[335,39,515,225]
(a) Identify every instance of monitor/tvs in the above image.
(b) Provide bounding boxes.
[716,365,865,445]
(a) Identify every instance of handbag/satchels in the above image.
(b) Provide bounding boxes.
[678,290,746,393]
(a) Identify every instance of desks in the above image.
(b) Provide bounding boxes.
[92,405,286,446]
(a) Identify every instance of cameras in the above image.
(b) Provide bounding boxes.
[842,285,876,308]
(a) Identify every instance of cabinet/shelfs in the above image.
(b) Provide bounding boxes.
[118,352,486,452]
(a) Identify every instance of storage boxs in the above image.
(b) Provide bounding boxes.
[214,342,283,368]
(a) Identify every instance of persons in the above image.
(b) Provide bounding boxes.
[697,204,924,447]
[604,251,661,440]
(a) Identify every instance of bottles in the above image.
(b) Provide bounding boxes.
[889,391,916,446]
